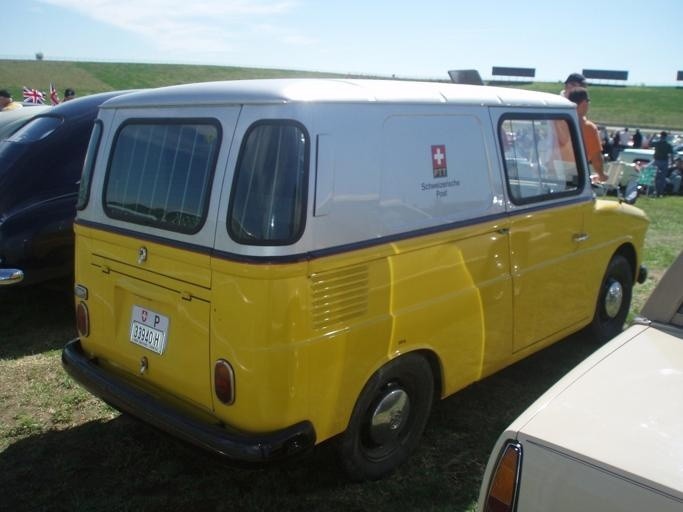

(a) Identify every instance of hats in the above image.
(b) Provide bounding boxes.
[564,73,593,85]
[65,89,75,96]
[0,89,14,98]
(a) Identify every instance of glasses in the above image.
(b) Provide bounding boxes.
[587,98,592,104]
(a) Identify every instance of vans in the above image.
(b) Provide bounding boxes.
[61,76,652,482]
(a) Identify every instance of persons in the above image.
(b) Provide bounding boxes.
[501,73,683,198]
[61,87,76,104]
[0,89,23,111]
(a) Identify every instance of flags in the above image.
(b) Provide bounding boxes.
[49,83,59,106]
[23,86,46,104]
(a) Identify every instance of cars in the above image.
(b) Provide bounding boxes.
[0,87,155,288]
[595,123,683,157]
